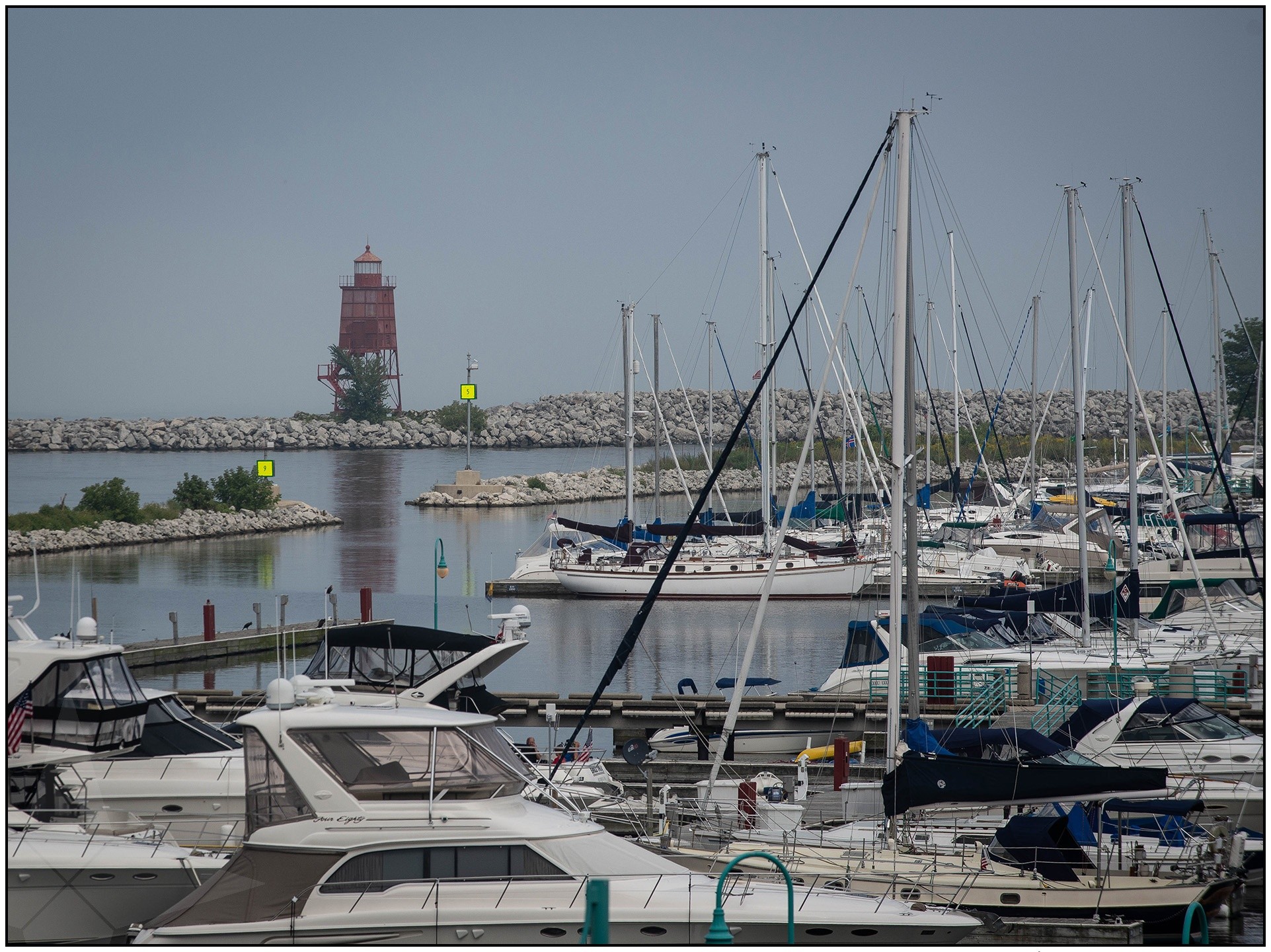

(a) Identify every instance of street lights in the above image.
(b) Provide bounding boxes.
[434,537,449,629]
[465,350,479,466]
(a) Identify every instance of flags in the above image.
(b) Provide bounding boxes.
[577,728,593,763]
[8,683,33,755]
[981,848,988,870]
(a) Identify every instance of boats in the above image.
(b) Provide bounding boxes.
[7,546,985,945]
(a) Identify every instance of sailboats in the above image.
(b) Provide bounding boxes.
[485,91,1265,946]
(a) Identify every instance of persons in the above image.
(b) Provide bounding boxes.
[526,737,541,760]
[564,740,580,762]
[551,747,565,763]
